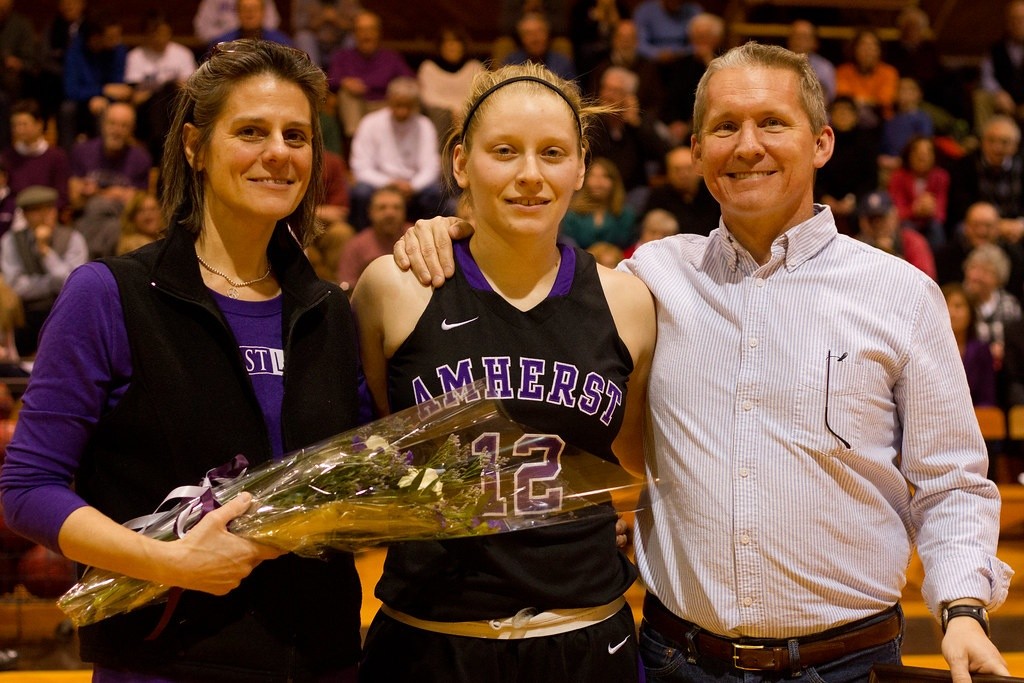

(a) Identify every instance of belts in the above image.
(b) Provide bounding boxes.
[643,594,902,673]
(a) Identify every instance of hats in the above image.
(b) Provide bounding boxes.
[17,186,59,205]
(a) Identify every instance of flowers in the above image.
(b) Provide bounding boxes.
[50,431,506,629]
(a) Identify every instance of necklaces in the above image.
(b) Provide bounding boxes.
[196,253,272,286]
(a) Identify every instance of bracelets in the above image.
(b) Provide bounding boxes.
[939,604,996,637]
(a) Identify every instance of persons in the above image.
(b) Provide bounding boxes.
[0,0,1024,683]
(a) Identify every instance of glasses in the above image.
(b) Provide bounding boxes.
[825,349,851,449]
[206,41,312,63]
